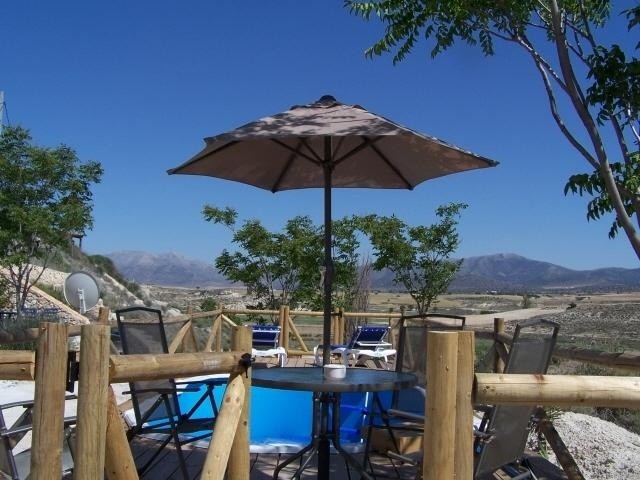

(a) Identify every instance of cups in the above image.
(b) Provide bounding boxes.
[322,364,347,382]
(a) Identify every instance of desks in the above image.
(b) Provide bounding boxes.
[252,367,417,480]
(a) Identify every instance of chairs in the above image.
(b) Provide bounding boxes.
[386,319,560,480]
[314,326,397,371]
[250,325,288,367]
[115,307,224,480]
[358,315,466,480]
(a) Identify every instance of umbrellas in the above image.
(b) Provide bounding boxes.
[165,94,500,373]
